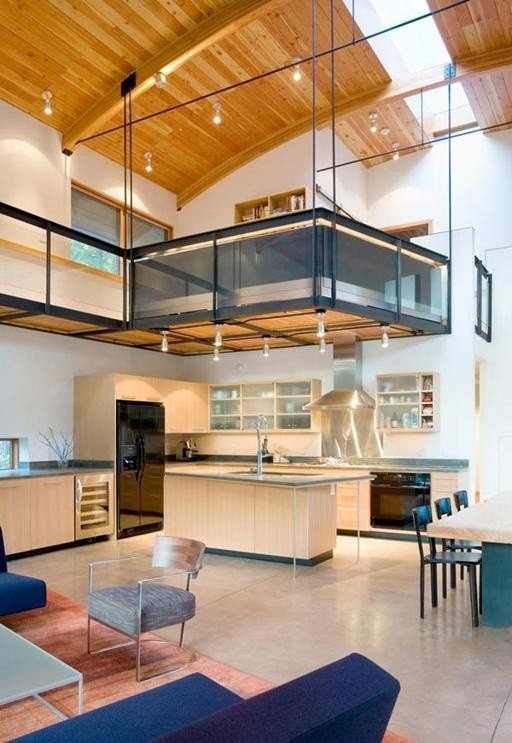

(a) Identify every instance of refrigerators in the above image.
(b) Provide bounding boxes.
[115,401,164,539]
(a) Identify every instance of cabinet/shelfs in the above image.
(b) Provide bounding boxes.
[71,372,166,471]
[232,183,314,253]
[367,368,443,437]
[164,377,209,435]
[205,375,323,436]
[0,466,76,562]
[165,462,471,541]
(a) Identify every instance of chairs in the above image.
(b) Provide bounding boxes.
[408,488,483,628]
[86,531,208,684]
[0,525,47,620]
[3,649,401,743]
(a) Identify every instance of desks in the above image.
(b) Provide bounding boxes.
[0,619,86,742]
[166,468,379,584]
[424,487,510,633]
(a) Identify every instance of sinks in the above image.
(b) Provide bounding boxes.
[219,470,325,476]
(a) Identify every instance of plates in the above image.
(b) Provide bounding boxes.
[285,402,295,412]
[422,406,432,413]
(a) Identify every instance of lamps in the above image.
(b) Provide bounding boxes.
[39,56,402,175]
[209,307,390,363]
[158,326,171,353]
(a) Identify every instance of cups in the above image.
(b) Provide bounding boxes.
[378,394,411,403]
[214,403,223,414]
[231,390,238,398]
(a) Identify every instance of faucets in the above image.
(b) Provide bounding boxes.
[256,415,268,473]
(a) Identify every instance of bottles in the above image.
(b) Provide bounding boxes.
[378,407,418,428]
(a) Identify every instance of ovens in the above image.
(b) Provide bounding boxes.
[370,471,432,529]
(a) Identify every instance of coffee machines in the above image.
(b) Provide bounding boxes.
[175,440,194,461]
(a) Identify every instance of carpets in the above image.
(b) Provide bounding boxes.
[1,587,417,742]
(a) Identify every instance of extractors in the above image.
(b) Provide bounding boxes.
[302,334,377,411]
